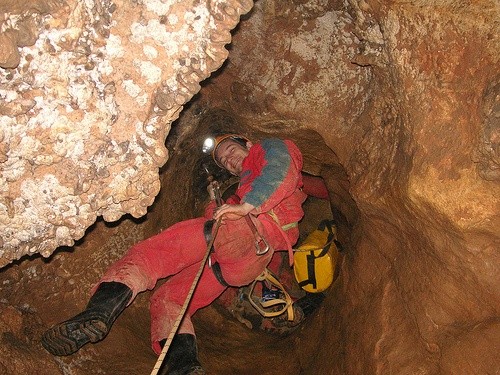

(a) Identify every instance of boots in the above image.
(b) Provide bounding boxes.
[159,333,204,375]
[41,281,133,356]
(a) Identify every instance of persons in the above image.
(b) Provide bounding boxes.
[218,174,329,331]
[40,134,307,375]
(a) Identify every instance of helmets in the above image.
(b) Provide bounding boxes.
[211,134,252,170]
